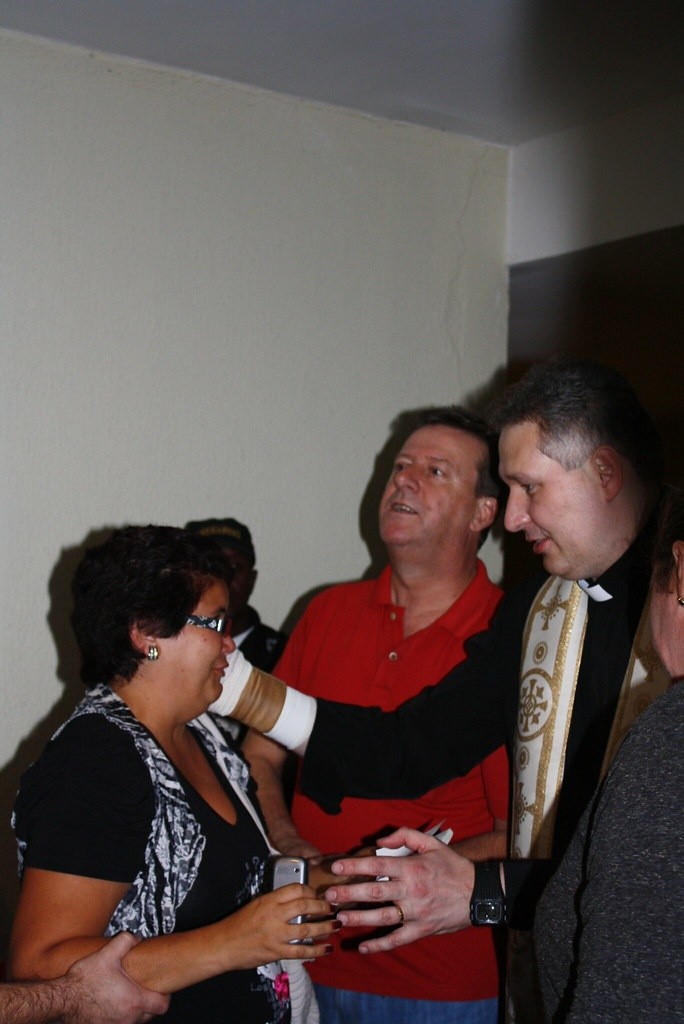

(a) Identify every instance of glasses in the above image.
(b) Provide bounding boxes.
[182,615,233,637]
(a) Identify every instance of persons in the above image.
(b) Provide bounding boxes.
[0,516,370,1024]
[211,352,684,1024]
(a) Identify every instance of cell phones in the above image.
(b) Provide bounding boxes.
[273,857,309,945]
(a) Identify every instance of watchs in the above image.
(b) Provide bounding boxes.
[470,862,506,927]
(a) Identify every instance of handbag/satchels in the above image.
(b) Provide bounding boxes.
[280,959,321,1024]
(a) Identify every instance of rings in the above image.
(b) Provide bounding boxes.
[395,904,404,923]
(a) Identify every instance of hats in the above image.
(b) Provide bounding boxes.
[183,518,256,568]
